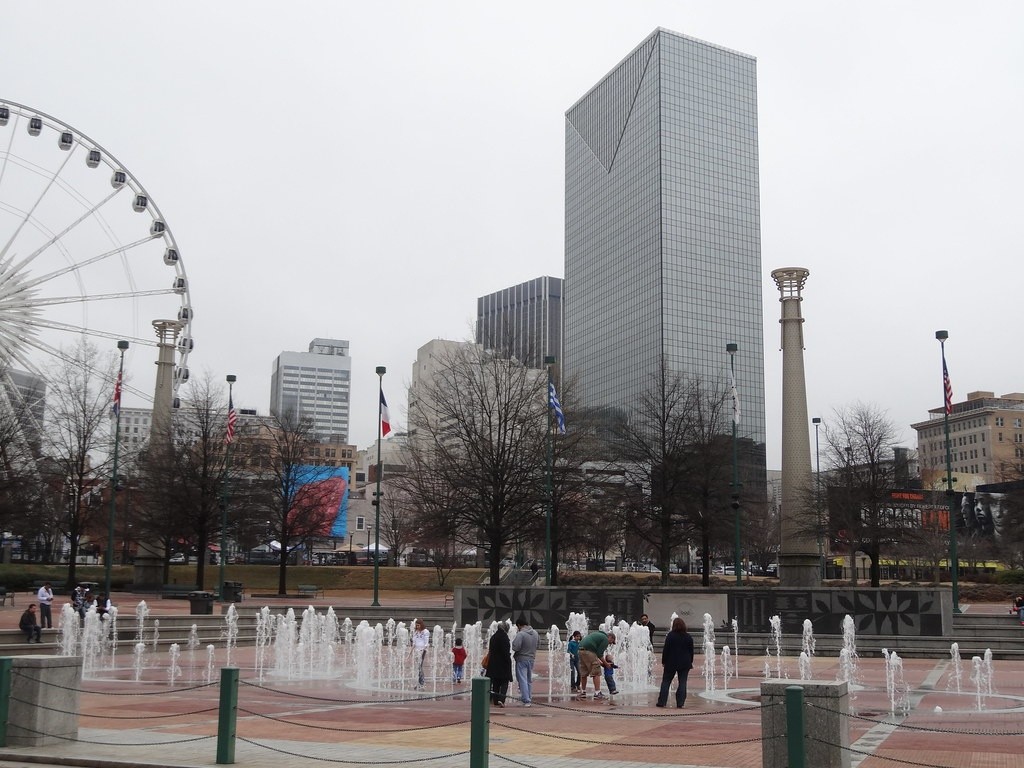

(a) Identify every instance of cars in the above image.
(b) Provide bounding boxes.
[714,565,752,577]
[170,552,187,564]
[602,562,660,572]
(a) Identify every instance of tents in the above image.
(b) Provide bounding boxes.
[335,543,389,552]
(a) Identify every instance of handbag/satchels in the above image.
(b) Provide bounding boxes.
[481,652,490,669]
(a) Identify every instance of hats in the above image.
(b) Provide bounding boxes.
[609,633,616,644]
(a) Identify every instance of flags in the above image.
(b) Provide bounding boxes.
[113,362,123,417]
[943,355,953,416]
[379,388,391,437]
[549,372,566,436]
[731,373,740,424]
[224,395,236,445]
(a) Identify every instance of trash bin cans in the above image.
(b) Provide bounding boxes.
[827,566,836,578]
[615,556,623,571]
[78,581,99,595]
[586,557,596,570]
[223,581,243,602]
[835,566,842,579]
[189,591,214,614]
[347,551,356,565]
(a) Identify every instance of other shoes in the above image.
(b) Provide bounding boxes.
[677,705,682,708]
[580,691,586,698]
[27,638,34,644]
[656,703,664,707]
[36,638,45,643]
[498,701,505,707]
[456,677,461,684]
[517,702,532,707]
[611,690,619,694]
[593,690,605,699]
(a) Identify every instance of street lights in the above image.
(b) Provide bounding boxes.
[366,524,372,564]
[348,531,353,563]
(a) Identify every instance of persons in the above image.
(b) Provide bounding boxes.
[450,637,468,684]
[636,613,656,651]
[655,618,694,710]
[18,604,44,644]
[510,617,540,707]
[530,559,540,583]
[960,491,1016,548]
[407,618,430,690]
[304,552,345,567]
[70,583,112,622]
[1013,596,1024,625]
[566,630,621,700]
[486,622,513,707]
[37,582,54,629]
